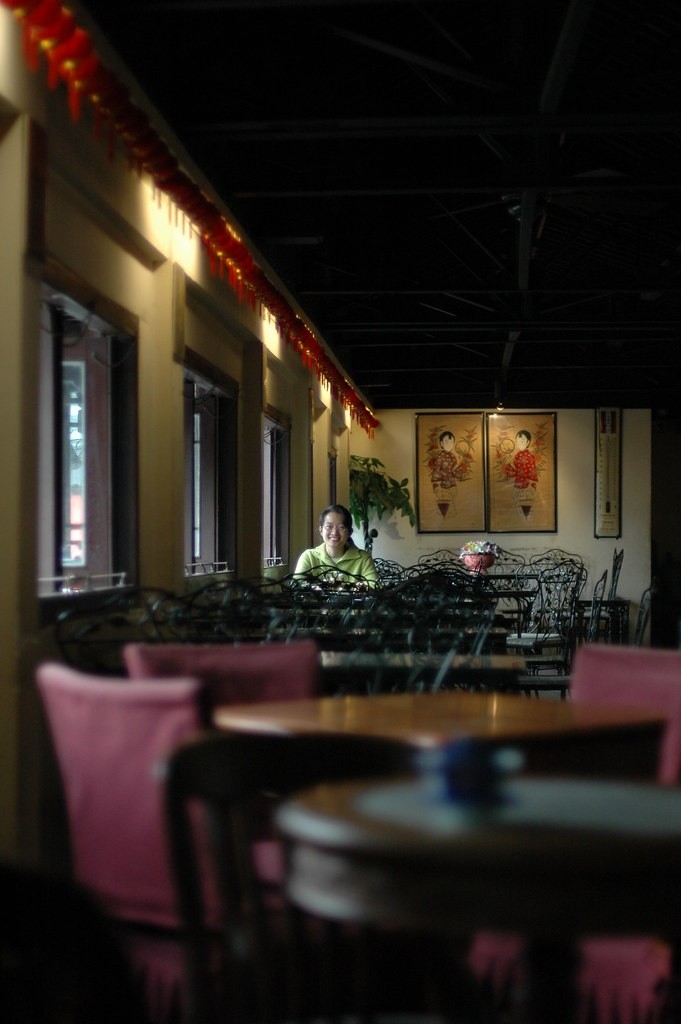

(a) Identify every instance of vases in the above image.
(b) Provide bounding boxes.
[464,553,495,575]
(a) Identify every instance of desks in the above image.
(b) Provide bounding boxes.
[318,650,526,667]
[215,695,669,784]
[274,771,681,938]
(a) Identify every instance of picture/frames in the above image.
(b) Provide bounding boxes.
[414,412,487,534]
[486,411,557,532]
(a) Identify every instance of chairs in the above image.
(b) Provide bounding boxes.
[0,549,681,1024]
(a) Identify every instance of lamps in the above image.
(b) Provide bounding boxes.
[497,400,505,410]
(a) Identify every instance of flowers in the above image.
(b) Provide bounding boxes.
[459,540,500,559]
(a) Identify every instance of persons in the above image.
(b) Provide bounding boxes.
[291,504,383,590]
